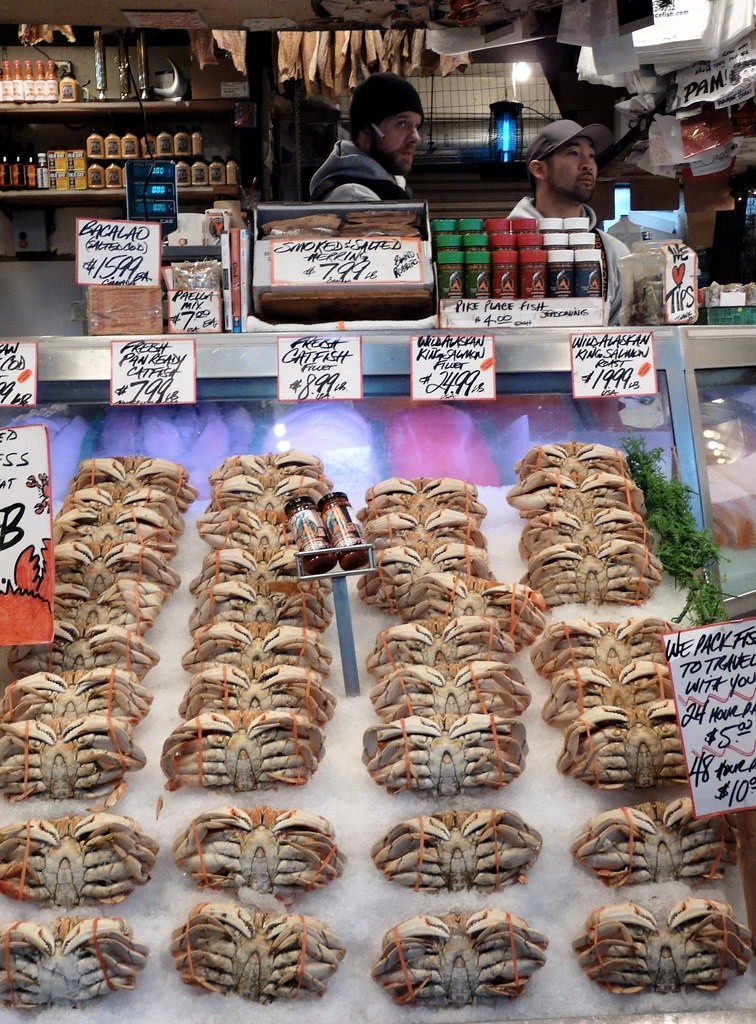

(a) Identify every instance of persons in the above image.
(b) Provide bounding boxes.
[482,119,635,327]
[308,71,425,203]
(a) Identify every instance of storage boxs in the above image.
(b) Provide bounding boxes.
[47,149,87,190]
[86,285,164,335]
[694,305,756,325]
[191,58,252,100]
[253,198,435,312]
[168,289,223,332]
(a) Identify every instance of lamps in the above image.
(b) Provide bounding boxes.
[488,100,524,165]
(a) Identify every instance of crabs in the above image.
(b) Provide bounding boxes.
[0,442,751,1006]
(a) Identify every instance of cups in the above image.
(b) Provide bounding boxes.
[74,80,89,102]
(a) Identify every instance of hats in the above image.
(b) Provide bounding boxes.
[526,119,613,164]
[349,74,424,132]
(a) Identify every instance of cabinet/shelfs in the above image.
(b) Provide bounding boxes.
[0,99,238,208]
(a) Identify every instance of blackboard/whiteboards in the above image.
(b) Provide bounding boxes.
[163,266,222,332]
[0,341,36,407]
[0,423,56,646]
[110,338,198,405]
[570,331,659,400]
[661,617,756,819]
[276,337,365,401]
[268,237,423,286]
[75,217,162,287]
[662,241,699,326]
[410,333,495,401]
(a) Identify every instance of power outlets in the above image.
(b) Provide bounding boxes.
[52,60,72,73]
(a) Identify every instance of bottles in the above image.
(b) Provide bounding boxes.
[139,127,156,159]
[0,60,58,103]
[59,72,82,102]
[175,156,238,186]
[86,127,104,159]
[121,127,140,159]
[0,142,38,190]
[192,126,203,155]
[37,153,48,190]
[87,161,127,189]
[104,127,121,158]
[174,127,191,156]
[607,215,643,254]
[431,218,603,299]
[317,492,369,571]
[156,127,174,155]
[283,496,336,575]
